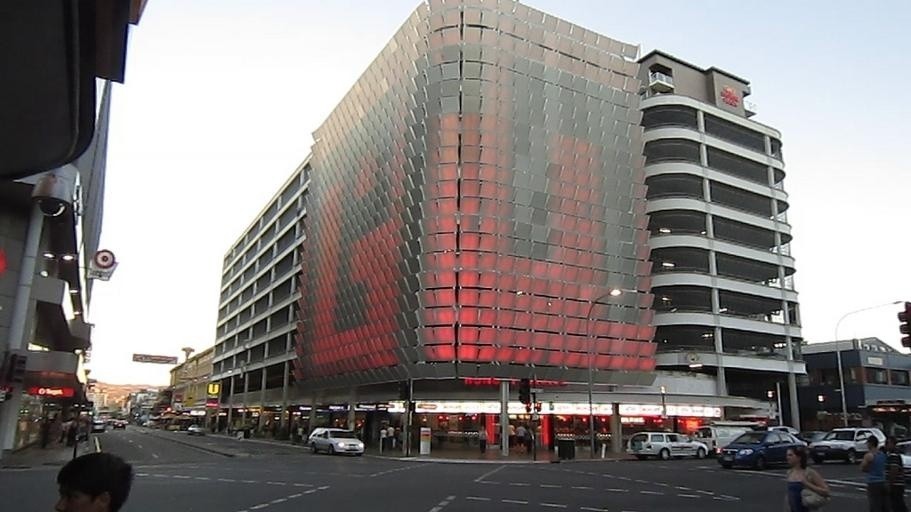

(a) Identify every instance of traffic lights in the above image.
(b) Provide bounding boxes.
[897,301,910,348]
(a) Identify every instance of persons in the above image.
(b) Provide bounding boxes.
[55,453,134,511]
[377,422,404,452]
[861,434,908,511]
[477,422,535,457]
[783,445,833,512]
[295,424,304,443]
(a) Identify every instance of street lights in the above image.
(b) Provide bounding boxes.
[767,390,775,427]
[832,300,905,431]
[660,385,669,433]
[586,287,624,461]
[817,394,825,433]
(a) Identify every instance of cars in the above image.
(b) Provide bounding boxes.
[716,428,809,471]
[186,425,205,437]
[884,440,911,480]
[797,430,829,446]
[308,427,365,457]
[91,420,106,433]
[113,420,126,430]
[768,425,800,438]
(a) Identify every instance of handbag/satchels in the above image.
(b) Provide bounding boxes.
[800,487,830,507]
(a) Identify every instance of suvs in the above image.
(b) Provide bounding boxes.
[809,426,888,466]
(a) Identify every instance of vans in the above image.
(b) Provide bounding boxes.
[692,424,755,456]
[625,430,710,460]
